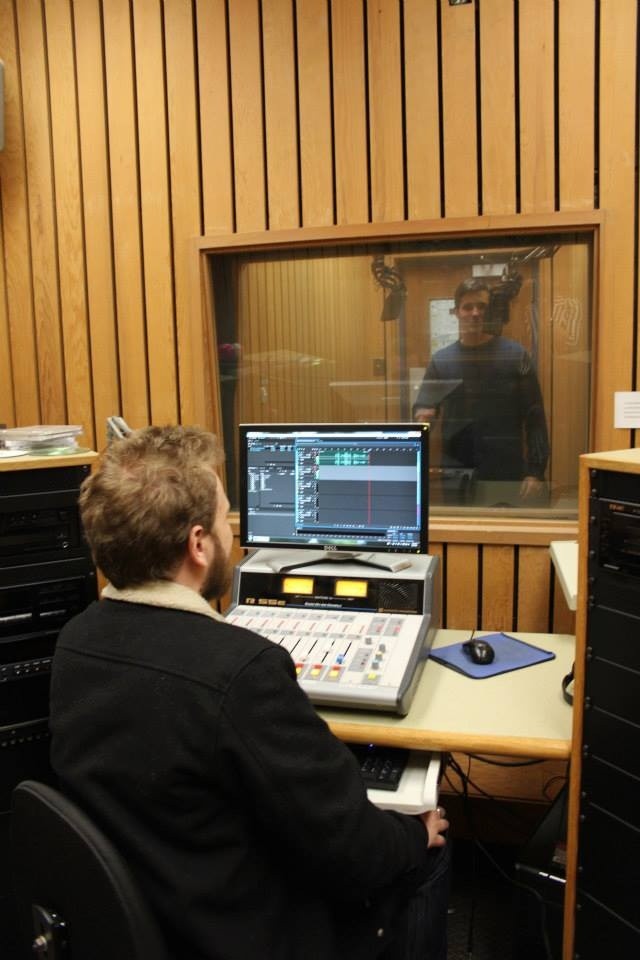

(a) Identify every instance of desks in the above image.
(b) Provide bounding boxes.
[314,628,577,757]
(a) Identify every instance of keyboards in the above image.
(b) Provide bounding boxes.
[347,744,411,792]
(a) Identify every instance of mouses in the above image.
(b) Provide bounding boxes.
[464,640,496,665]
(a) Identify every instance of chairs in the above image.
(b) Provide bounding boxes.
[6,781,447,960]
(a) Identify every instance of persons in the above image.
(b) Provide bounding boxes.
[48,425,456,960]
[412,276,549,509]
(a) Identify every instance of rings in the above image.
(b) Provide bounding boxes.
[437,809,443,818]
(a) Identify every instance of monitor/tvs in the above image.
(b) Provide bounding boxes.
[238,425,428,553]
